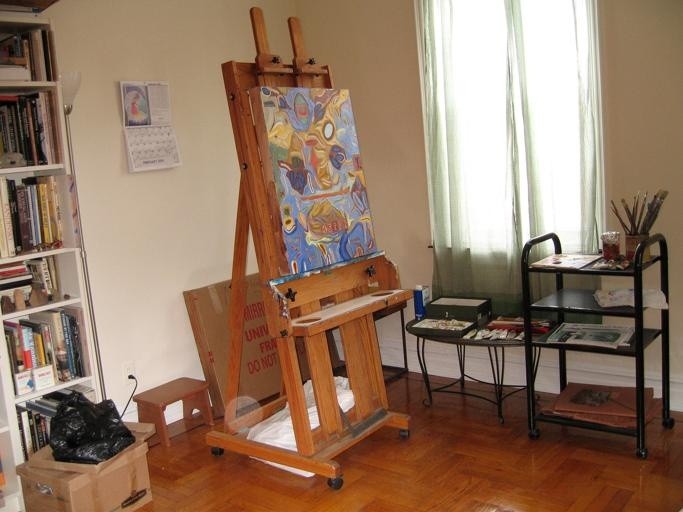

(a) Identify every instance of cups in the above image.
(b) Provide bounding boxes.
[626,235,651,265]
[601,232,620,259]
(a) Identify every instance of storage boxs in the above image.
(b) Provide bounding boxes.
[16,442,153,512]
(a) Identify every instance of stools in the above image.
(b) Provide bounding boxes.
[134,378,215,447]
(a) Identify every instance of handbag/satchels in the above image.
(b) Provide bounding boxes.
[48,392,136,464]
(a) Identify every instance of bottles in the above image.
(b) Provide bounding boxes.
[413,285,424,320]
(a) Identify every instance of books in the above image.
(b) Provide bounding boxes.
[539,381,665,431]
[617,326,635,346]
[0,175,64,307]
[593,288,669,311]
[0,28,60,82]
[0,91,62,169]
[3,305,91,463]
[545,322,630,349]
[530,253,603,270]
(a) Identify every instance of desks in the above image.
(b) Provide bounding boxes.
[407,317,556,423]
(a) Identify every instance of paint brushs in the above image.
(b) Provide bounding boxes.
[610,188,671,235]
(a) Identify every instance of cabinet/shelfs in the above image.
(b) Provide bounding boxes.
[1,11,103,512]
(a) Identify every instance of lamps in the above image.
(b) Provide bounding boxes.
[61,68,106,401]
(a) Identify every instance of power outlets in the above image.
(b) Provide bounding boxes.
[121,360,137,385]
